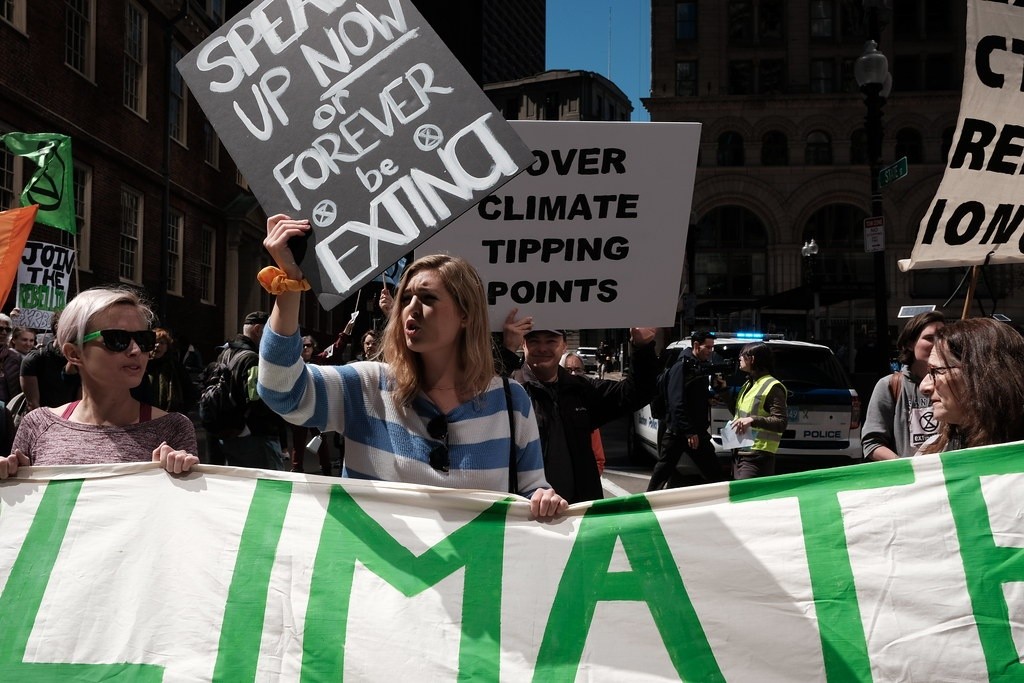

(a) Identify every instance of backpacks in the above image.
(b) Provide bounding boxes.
[650,356,714,420]
[199,347,258,440]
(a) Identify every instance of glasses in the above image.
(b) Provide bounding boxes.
[562,367,584,374]
[74,327,157,352]
[927,365,966,385]
[363,341,379,346]
[155,340,168,345]
[303,343,314,348]
[0,326,13,332]
[427,412,449,471]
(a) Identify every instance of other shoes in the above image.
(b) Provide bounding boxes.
[289,467,305,473]
[283,452,290,460]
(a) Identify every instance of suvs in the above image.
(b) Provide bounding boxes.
[575,346,598,371]
[633,331,864,478]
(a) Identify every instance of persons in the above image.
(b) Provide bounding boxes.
[495,307,660,504]
[713,341,790,479]
[256,212,569,520]
[912,316,1024,457]
[0,284,201,482]
[860,310,950,462]
[0,307,607,503]
[646,329,724,492]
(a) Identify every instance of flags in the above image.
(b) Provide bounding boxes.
[1,130,78,237]
[0,203,41,313]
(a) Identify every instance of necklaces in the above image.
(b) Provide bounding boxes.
[419,384,456,391]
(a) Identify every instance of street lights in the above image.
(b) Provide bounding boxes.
[801,238,818,342]
[853,37,893,379]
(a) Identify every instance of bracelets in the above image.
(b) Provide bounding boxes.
[256,266,311,296]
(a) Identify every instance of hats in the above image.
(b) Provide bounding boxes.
[523,329,567,339]
[243,310,269,324]
